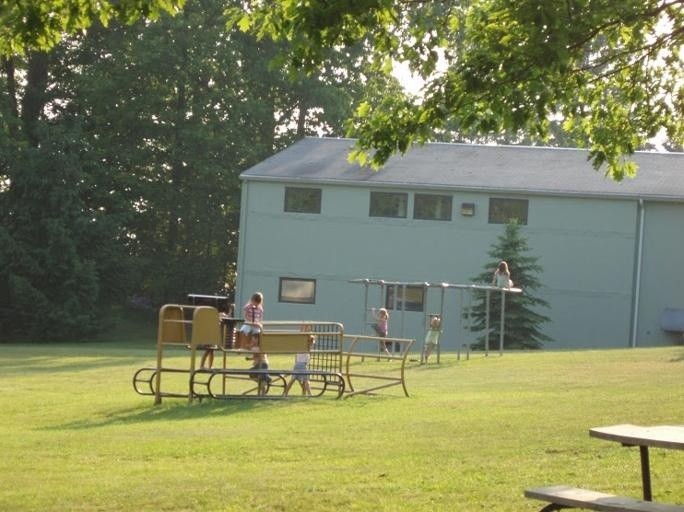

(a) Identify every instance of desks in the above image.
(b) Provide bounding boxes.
[589,422,684,502]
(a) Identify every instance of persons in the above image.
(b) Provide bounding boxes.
[199,299,229,370]
[423,314,441,364]
[490,261,513,290]
[283,336,315,397]
[370,307,392,362]
[237,291,272,396]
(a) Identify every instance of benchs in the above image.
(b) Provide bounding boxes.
[522,484,683,512]
